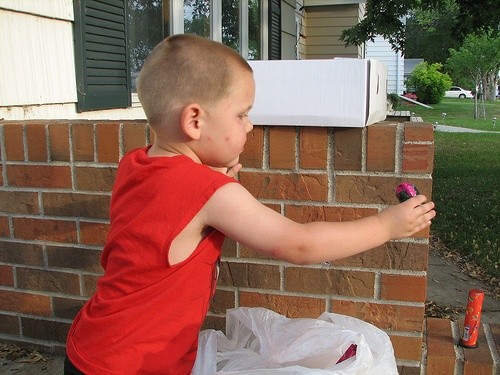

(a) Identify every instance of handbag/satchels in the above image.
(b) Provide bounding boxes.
[191,306,400,375]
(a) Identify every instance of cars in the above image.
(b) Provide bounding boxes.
[443,86,475,99]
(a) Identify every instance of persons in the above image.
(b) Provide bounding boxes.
[63,33,435,374]
